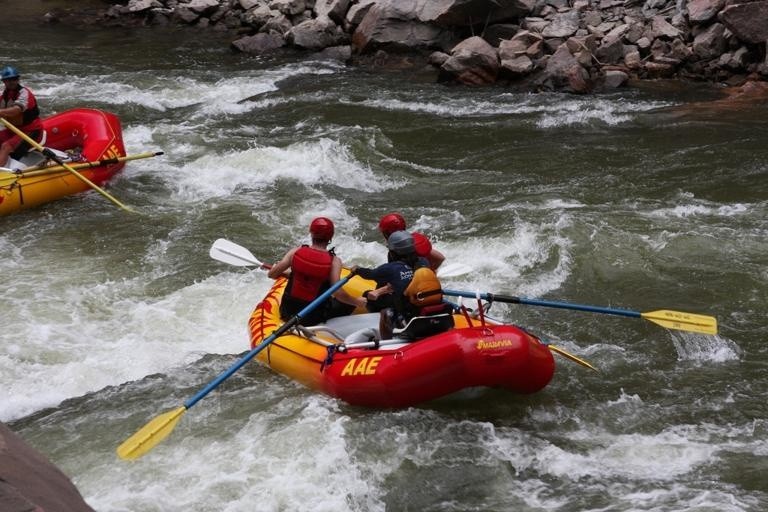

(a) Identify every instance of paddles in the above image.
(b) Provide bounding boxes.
[448,302,596,379]
[116,271,354,460]
[0,151,164,187]
[440,288,716,336]
[208,238,290,279]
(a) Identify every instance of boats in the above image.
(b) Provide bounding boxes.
[249,267,555,407]
[0,108,127,215]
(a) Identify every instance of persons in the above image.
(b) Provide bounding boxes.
[362,213,445,312]
[349,230,443,339]
[268,217,368,327]
[0,65,43,168]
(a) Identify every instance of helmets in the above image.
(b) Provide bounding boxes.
[309,217,333,242]
[1,66,20,80]
[379,214,405,232]
[388,231,415,255]
